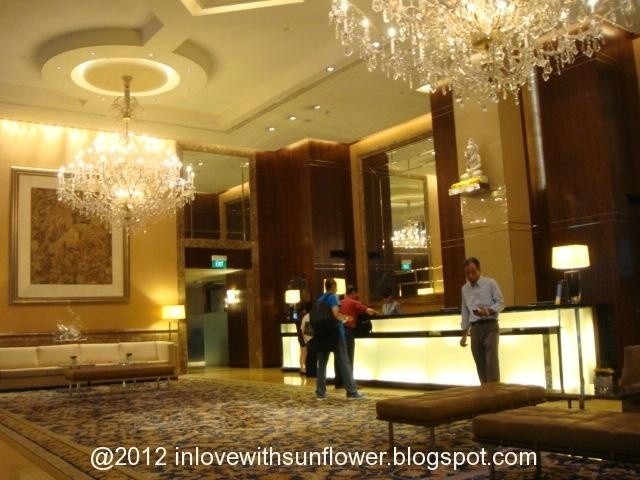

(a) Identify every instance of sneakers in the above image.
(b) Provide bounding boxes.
[347,390,364,397]
[316,391,326,398]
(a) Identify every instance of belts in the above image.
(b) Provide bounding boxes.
[471,318,496,325]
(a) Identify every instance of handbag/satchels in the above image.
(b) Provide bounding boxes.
[304,322,314,335]
[357,313,372,331]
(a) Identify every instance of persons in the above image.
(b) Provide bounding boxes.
[295,278,382,399]
[458,256,507,384]
[382,288,401,316]
[459,137,484,181]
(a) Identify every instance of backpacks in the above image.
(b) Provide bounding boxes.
[309,292,339,335]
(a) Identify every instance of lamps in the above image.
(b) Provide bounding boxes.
[323,277,346,298]
[282,278,307,323]
[390,198,432,250]
[417,287,435,295]
[324,0,637,115]
[52,72,198,237]
[550,244,591,306]
[161,302,188,341]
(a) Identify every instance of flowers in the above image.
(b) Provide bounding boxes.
[591,362,615,376]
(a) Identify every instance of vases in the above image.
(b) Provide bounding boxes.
[594,376,613,389]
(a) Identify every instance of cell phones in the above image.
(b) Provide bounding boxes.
[473,309,480,313]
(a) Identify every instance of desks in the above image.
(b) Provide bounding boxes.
[54,361,178,394]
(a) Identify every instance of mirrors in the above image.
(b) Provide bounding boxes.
[349,112,447,315]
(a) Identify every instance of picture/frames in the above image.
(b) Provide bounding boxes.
[5,165,130,310]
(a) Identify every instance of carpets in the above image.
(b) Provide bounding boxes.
[0,374,639,480]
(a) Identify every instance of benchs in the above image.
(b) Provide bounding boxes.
[373,381,546,457]
[470,404,640,478]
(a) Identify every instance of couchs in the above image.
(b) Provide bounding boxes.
[0,337,180,392]
